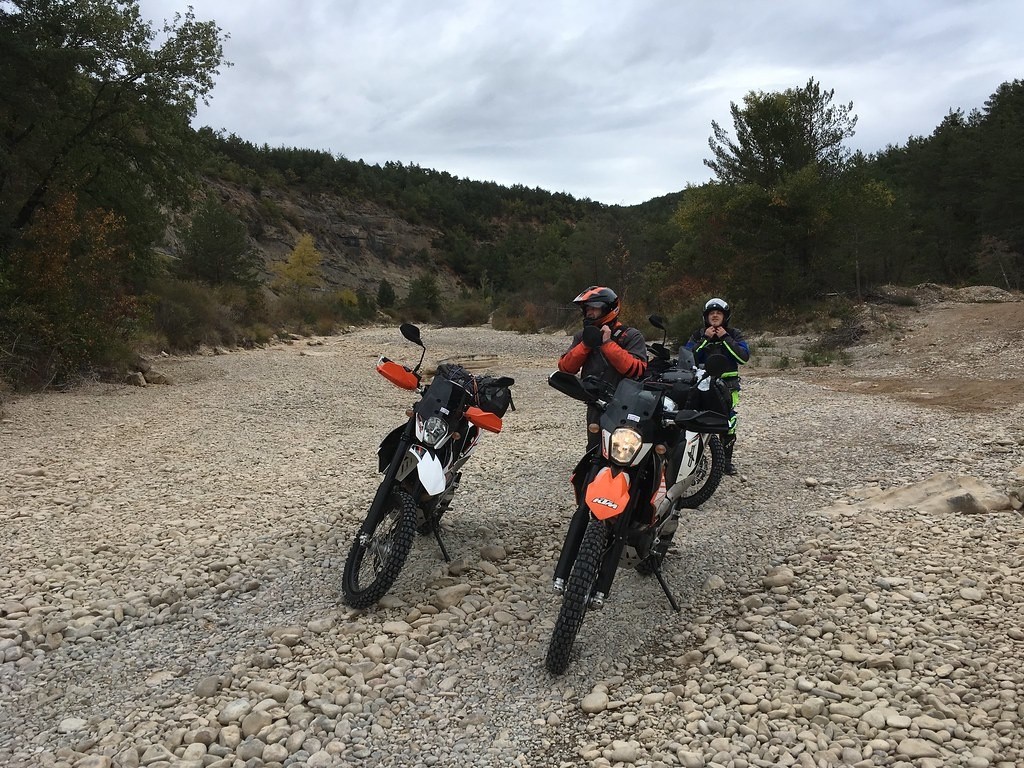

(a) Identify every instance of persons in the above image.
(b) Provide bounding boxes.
[686,298,750,475]
[558,285,647,453]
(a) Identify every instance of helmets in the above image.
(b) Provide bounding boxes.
[574,286,621,327]
[703,297,732,329]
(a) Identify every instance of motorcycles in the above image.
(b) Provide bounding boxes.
[645,314,733,509]
[545,326,729,672]
[341,322,516,610]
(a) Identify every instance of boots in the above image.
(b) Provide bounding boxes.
[721,434,737,474]
